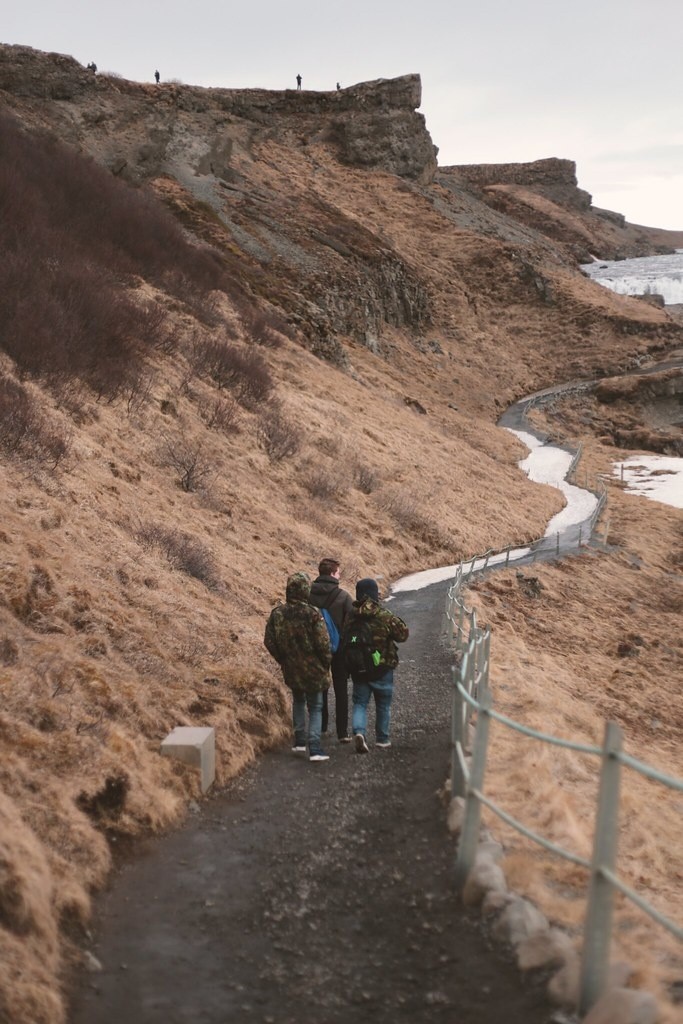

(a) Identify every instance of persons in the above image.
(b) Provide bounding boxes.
[87,62,96,72]
[337,83,340,89]
[296,74,302,89]
[305,558,408,753]
[155,70,160,84]
[264,572,332,761]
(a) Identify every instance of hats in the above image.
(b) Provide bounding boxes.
[355,579,379,601]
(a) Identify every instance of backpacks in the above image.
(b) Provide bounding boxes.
[341,612,387,682]
[319,588,343,652]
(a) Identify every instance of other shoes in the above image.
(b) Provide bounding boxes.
[356,734,368,753]
[310,750,329,761]
[338,734,352,742]
[292,741,306,751]
[375,737,391,747]
